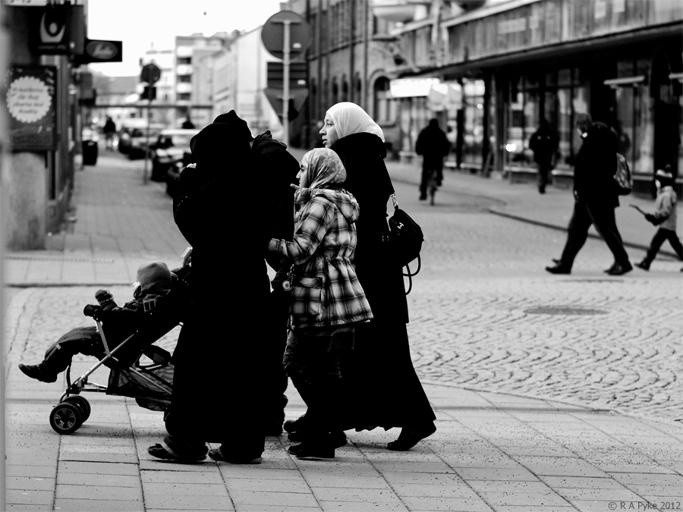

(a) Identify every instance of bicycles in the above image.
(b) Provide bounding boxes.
[428,160,437,205]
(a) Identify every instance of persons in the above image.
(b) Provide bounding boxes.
[545,114,635,277]
[550,121,629,274]
[634,164,683,271]
[415,117,451,204]
[17,258,168,382]
[528,118,559,194]
[145,100,437,464]
[101,116,116,150]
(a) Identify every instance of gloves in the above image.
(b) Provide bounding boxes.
[95,290,117,311]
[646,213,657,225]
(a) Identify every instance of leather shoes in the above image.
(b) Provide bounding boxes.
[546,258,571,273]
[18,364,57,383]
[388,423,436,450]
[284,420,347,458]
[148,444,207,459]
[209,449,262,464]
[608,261,632,275]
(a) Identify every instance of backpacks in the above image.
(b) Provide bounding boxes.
[608,152,633,195]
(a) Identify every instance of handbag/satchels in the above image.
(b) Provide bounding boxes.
[377,170,423,295]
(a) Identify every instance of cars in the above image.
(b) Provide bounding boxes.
[114,120,203,197]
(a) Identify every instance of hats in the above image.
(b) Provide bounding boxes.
[655,164,672,187]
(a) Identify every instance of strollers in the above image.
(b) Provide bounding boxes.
[46,246,192,433]
